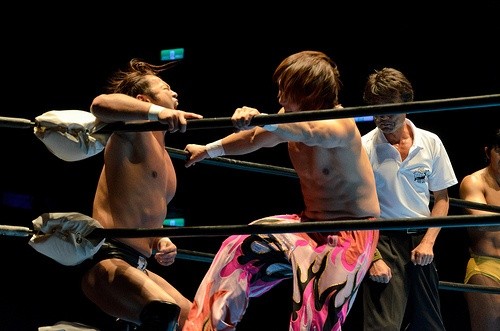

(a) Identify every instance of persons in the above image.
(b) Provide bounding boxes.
[460,130,500,331]
[82,61,204,331]
[180,51,382,331]
[361,68,459,331]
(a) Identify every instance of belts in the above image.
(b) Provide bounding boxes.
[396,229,423,236]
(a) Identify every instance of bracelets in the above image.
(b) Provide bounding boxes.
[205,139,226,159]
[260,113,278,132]
[148,105,164,122]
[371,248,382,262]
